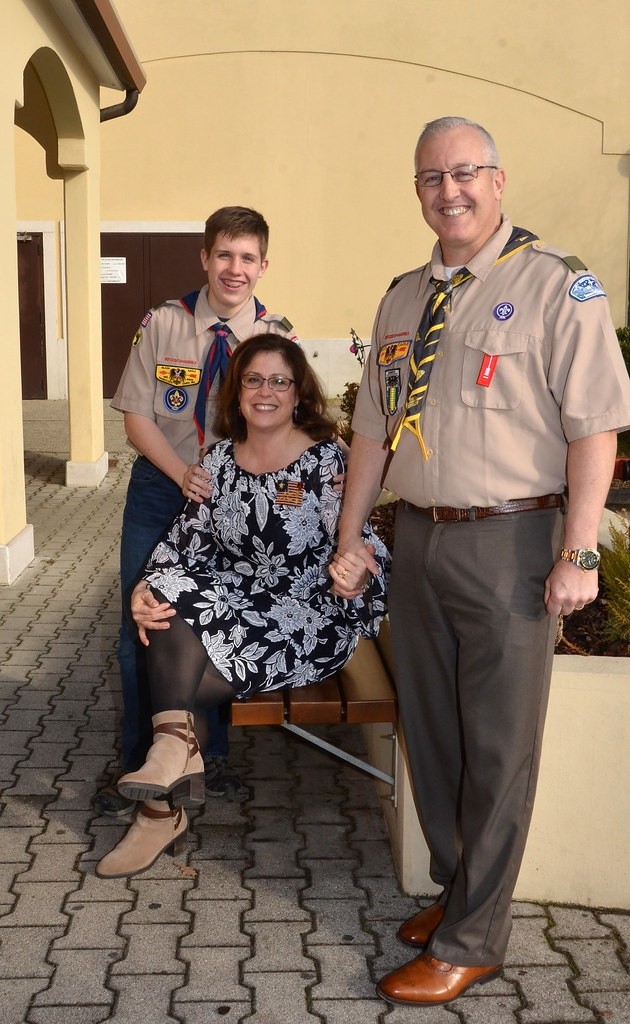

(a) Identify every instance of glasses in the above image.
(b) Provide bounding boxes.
[241,374,297,392]
[415,164,499,187]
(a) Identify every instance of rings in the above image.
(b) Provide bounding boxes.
[339,569,349,578]
[574,606,585,610]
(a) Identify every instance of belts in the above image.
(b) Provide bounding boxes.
[400,493,564,523]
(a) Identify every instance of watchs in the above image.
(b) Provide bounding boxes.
[558,547,602,573]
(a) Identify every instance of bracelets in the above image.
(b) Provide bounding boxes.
[360,569,372,594]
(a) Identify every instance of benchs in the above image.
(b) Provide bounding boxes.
[229,636,399,808]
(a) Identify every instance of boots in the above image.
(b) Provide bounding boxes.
[95,798,191,880]
[117,709,206,807]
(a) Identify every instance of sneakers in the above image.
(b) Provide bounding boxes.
[92,760,145,816]
[202,753,243,797]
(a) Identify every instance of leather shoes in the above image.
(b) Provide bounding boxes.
[375,949,505,1008]
[397,899,447,948]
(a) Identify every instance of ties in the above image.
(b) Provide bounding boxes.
[390,226,540,461]
[180,290,268,446]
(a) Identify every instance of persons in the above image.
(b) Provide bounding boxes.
[328,116,629,1008]
[85,206,351,817]
[94,332,393,879]
